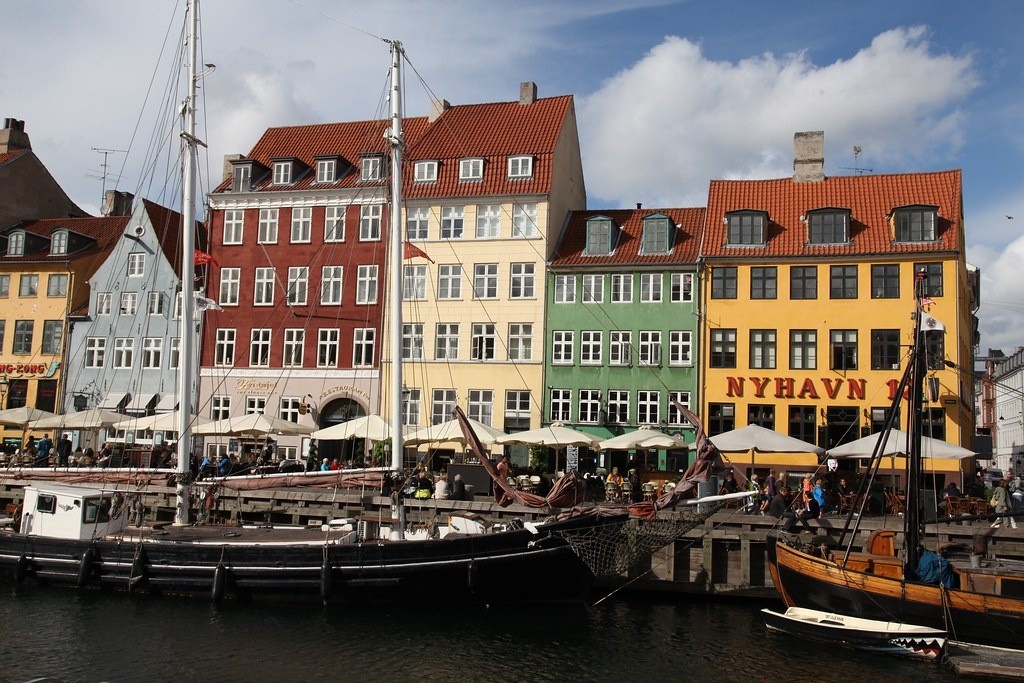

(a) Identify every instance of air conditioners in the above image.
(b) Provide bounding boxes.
[357,519,377,543]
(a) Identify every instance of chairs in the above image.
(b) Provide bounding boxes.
[946,495,989,525]
[605,481,674,504]
[507,471,565,496]
[838,493,871,515]
[884,492,906,517]
[0,452,93,469]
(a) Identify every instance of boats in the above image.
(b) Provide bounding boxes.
[761,606,951,666]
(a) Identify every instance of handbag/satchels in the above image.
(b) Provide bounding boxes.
[990,494,999,507]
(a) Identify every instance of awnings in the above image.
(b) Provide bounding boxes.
[96,392,128,412]
[125,394,155,413]
[154,395,179,414]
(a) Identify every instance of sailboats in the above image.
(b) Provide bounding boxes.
[765,267,1024,654]
[0,1,774,627]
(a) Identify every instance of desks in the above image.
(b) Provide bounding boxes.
[205,464,218,476]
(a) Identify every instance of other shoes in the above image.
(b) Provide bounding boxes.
[1013,525,1018,528]
[991,524,1001,528]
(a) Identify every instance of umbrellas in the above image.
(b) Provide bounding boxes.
[0,404,316,463]
[592,423,980,496]
[310,413,608,482]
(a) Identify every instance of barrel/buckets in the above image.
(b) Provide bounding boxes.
[968,555,983,569]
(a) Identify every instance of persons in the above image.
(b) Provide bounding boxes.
[938,461,1020,527]
[23,433,175,468]
[493,456,644,504]
[380,462,465,500]
[191,446,357,484]
[722,469,885,532]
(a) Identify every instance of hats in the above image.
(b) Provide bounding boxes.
[630,468,636,472]
